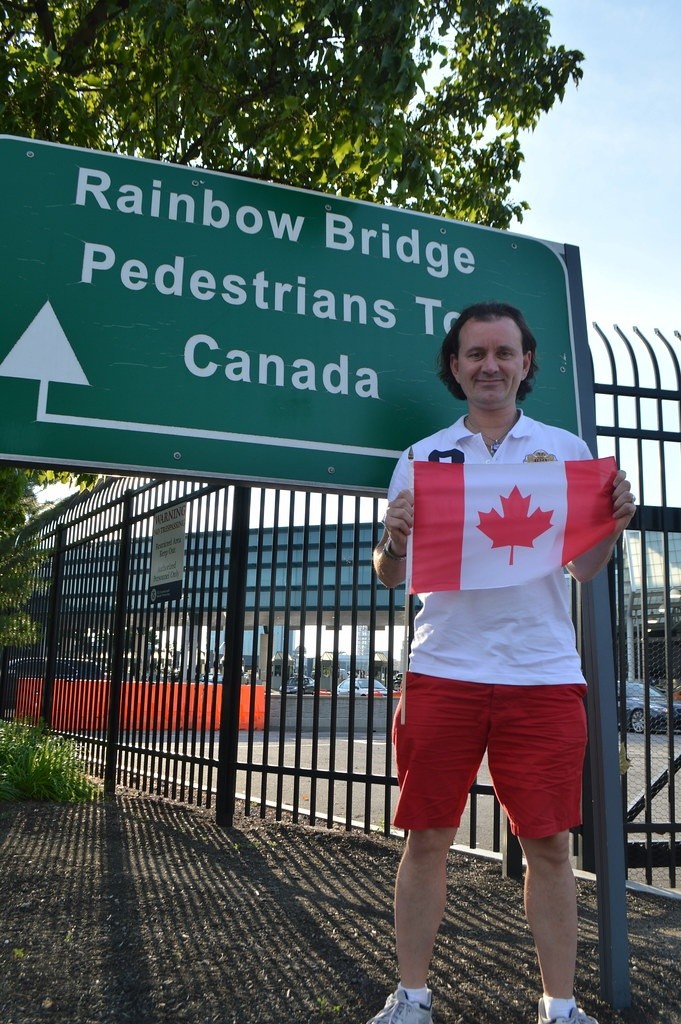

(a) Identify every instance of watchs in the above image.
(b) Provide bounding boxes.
[383,538,407,561]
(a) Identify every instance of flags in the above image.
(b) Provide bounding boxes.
[406,455,618,594]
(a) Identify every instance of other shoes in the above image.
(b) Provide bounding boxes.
[537,998,599,1024]
[366,989,434,1024]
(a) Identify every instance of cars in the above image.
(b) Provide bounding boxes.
[336,676,387,696]
[393,672,403,692]
[617,681,681,734]
[279,673,314,696]
[7,655,263,684]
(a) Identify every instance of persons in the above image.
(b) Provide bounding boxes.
[369,303,636,1024]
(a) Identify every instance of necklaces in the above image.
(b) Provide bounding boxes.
[466,417,517,452]
